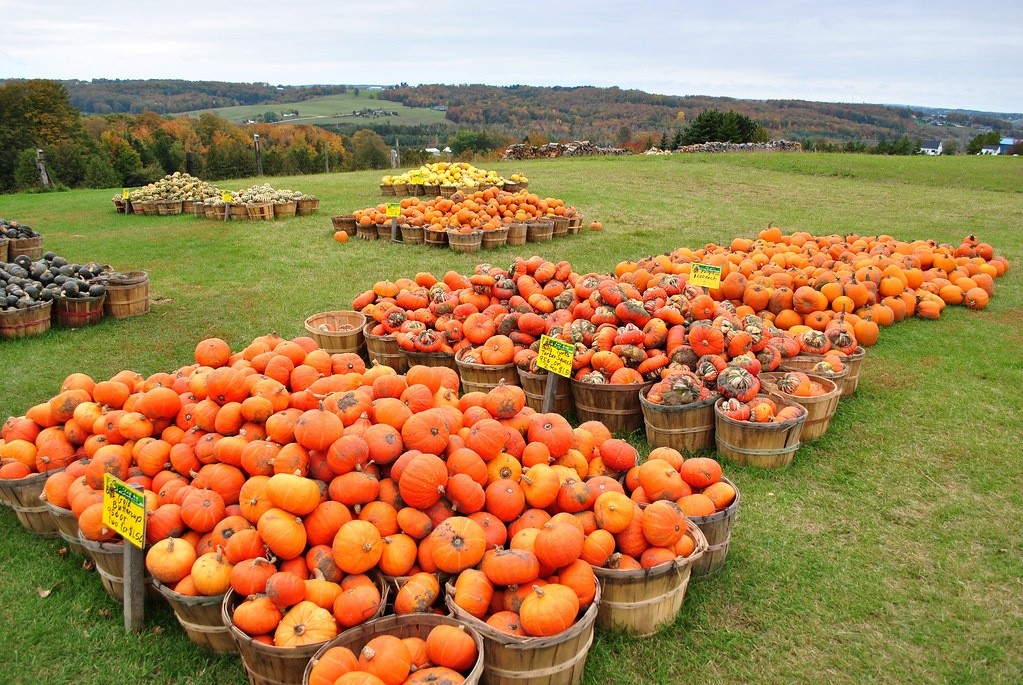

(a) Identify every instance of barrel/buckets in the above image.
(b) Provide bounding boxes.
[1,297,54,339]
[54,292,107,327]
[713,394,808,469]
[570,372,656,433]
[0,466,66,539]
[362,320,571,416]
[586,518,707,638]
[220,559,389,685]
[757,344,864,442]
[305,310,368,364]
[302,612,486,685]
[77,528,165,611]
[444,571,601,684]
[620,466,740,580]
[638,383,720,451]
[103,272,150,319]
[42,493,91,558]
[116,198,319,219]
[381,182,528,199]
[153,576,242,655]
[331,214,356,235]
[0,235,43,263]
[357,216,583,252]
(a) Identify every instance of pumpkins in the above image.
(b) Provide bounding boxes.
[0,162,1008,685]
